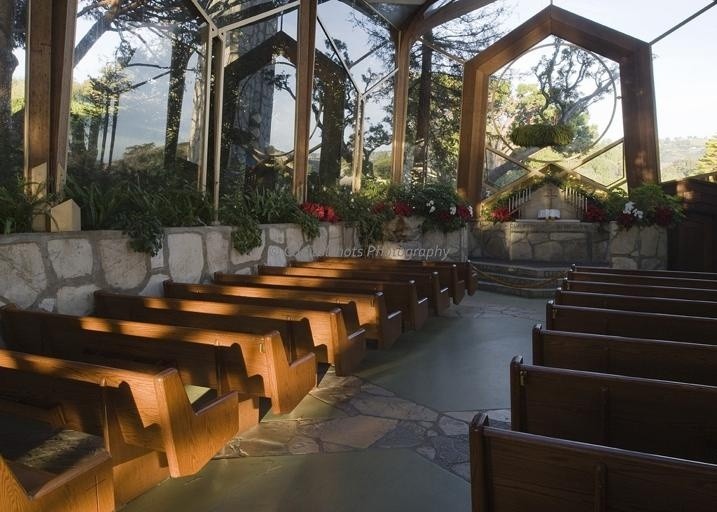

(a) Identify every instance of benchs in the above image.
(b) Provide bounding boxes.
[0,254,477,512]
[467,264,715,512]
